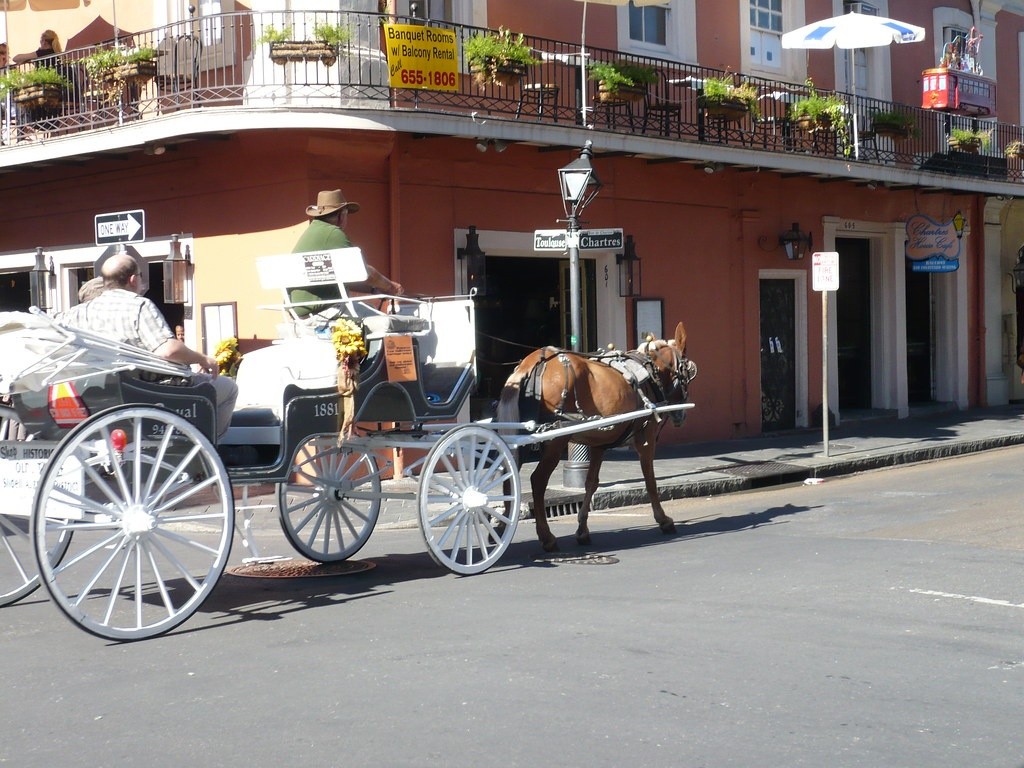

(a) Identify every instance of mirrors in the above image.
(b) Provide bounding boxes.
[200,300,239,355]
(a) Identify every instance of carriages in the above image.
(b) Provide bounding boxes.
[0,245,698,640]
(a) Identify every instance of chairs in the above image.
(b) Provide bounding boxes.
[81,86,111,130]
[641,65,682,138]
[592,77,636,132]
[57,59,88,134]
[750,111,791,151]
[811,122,838,156]
[515,68,563,122]
[850,104,883,163]
[151,31,207,114]
[705,109,747,146]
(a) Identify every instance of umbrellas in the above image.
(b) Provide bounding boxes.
[780,8,928,162]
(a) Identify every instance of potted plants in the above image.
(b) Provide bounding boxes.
[787,77,853,159]
[865,105,924,143]
[694,70,763,123]
[79,45,162,106]
[458,24,538,92]
[585,62,659,101]
[0,63,72,108]
[1003,139,1024,160]
[250,23,352,68]
[945,127,995,154]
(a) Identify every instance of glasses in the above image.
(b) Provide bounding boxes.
[130,272,142,278]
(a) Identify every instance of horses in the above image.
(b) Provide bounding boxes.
[487,320,690,554]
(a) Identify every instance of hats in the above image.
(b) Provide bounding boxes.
[305,189,360,216]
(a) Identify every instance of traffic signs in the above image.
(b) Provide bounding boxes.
[94,208,145,243]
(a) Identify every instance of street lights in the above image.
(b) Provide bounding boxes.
[556,136,603,486]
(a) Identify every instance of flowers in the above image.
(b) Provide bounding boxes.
[333,317,362,387]
[209,333,241,374]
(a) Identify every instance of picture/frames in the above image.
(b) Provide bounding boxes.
[632,297,664,349]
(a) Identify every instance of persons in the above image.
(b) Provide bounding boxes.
[0,42,16,119]
[289,189,402,335]
[62,243,239,454]
[13,29,79,101]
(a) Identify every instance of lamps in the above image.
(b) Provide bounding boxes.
[614,234,646,297]
[160,229,197,307]
[455,224,491,303]
[28,242,57,310]
[756,221,813,261]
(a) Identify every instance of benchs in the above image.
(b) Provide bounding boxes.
[250,245,434,399]
[234,323,391,434]
[6,348,231,472]
